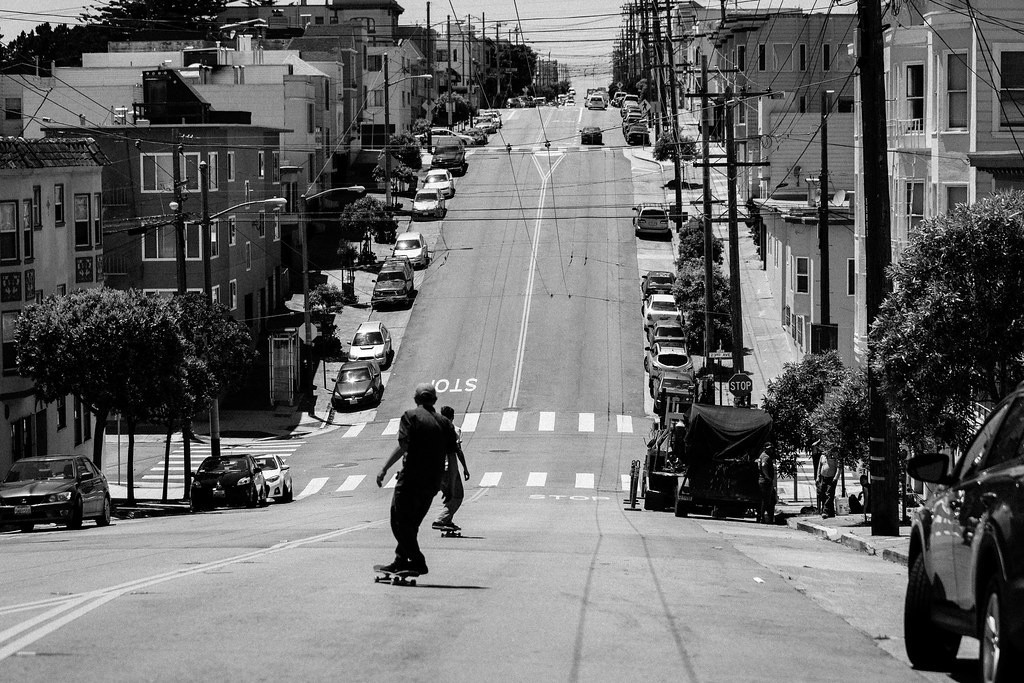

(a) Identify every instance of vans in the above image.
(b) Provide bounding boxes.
[391,231,429,268]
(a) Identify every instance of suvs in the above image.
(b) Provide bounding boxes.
[370,255,415,310]
[0,453,113,533]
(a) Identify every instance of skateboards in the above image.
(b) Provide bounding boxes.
[373,565,419,586]
[432,526,462,537]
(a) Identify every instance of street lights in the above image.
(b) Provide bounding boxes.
[299,184,366,416]
[197,159,289,457]
[381,52,433,208]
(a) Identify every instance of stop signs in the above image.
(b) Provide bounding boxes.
[728,373,753,397]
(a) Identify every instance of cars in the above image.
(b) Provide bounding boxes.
[640,271,677,296]
[186,453,267,512]
[408,187,445,221]
[346,320,393,369]
[632,202,671,237]
[414,86,651,176]
[898,379,1024,683]
[579,126,604,145]
[330,360,383,410]
[421,167,455,198]
[624,318,779,525]
[236,453,293,506]
[641,292,682,331]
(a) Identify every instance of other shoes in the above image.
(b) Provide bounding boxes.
[822,513,828,518]
[378,559,429,576]
[443,523,461,530]
[432,521,443,529]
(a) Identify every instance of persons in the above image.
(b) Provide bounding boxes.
[376,382,457,577]
[432,406,470,531]
[757,442,777,523]
[816,452,840,518]
[857,475,872,513]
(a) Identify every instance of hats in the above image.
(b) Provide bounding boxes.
[415,382,437,398]
